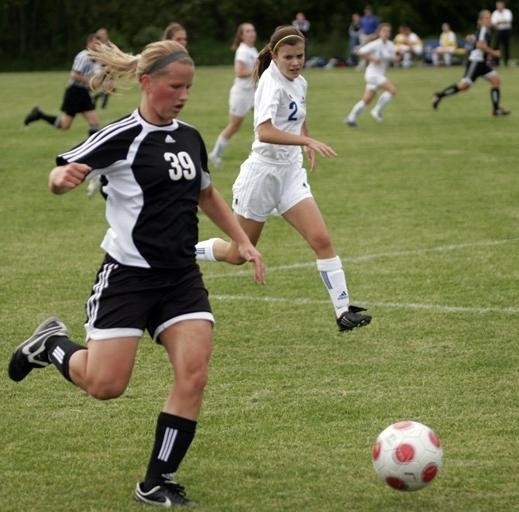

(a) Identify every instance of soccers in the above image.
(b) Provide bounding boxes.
[372,421,444,491]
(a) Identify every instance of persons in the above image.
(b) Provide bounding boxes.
[93,28,118,111]
[24,33,104,139]
[192,27,372,332]
[86,24,187,201]
[10,40,269,508]
[288,0,513,129]
[206,22,259,166]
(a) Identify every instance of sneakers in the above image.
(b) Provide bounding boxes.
[8,316,69,381]
[432,94,439,107]
[133,478,196,507]
[25,107,39,124]
[337,305,372,331]
[494,109,509,114]
